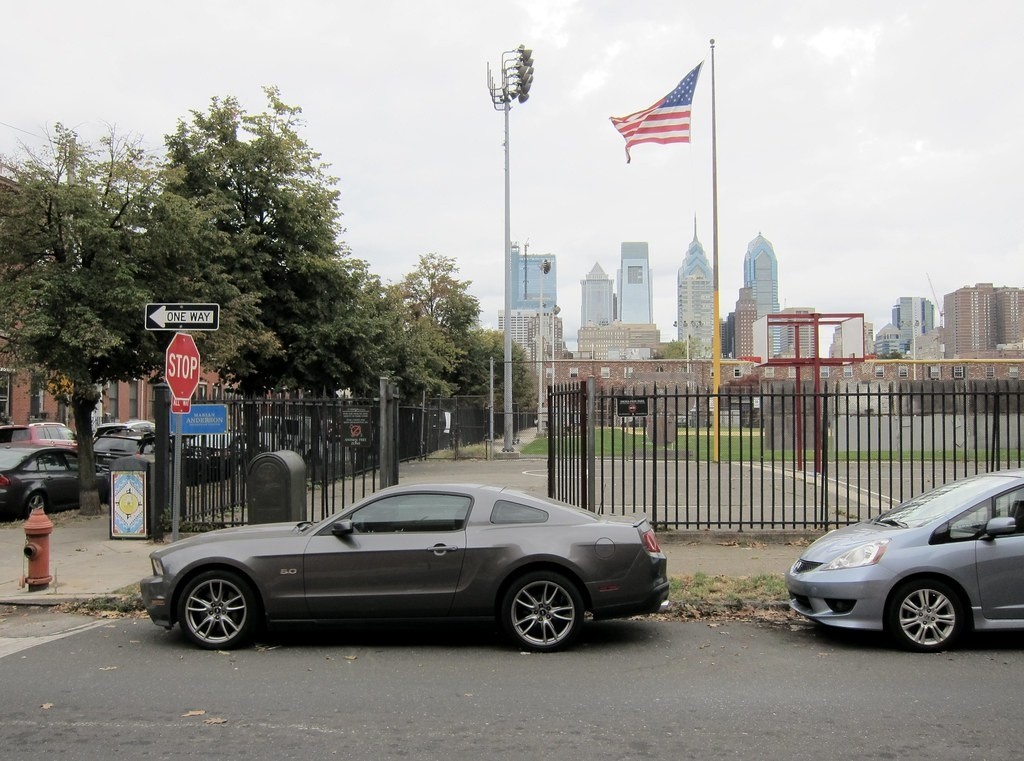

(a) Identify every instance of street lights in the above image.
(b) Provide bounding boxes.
[538,259,553,437]
[487,45,536,457]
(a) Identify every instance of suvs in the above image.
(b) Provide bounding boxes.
[0,421,79,448]
[93,421,156,437]
[93,427,156,473]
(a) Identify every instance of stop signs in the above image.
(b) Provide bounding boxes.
[165,333,201,401]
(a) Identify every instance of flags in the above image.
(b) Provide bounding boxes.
[608,60,703,163]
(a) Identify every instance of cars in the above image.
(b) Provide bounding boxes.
[183,432,269,482]
[0,442,109,515]
[139,482,668,650]
[781,465,1024,650]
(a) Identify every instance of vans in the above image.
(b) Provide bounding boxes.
[256,415,328,463]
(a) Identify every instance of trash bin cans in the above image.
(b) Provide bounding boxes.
[108,451,187,540]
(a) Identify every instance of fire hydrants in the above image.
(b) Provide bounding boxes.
[22,505,53,592]
